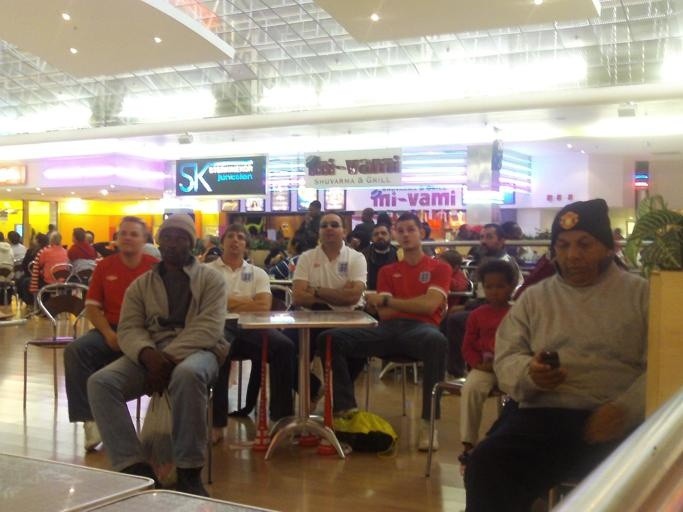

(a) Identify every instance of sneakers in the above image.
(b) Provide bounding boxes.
[84,421,102,451]
[418,428,438,450]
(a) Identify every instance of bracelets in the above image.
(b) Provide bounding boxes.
[313,286,321,297]
[383,293,387,307]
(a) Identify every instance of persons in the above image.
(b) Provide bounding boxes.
[330,211,452,453]
[86,213,228,498]
[202,223,272,448]
[346,207,525,297]
[62,215,161,452]
[144,234,159,257]
[456,257,520,466]
[195,235,222,262]
[269,213,367,422]
[463,198,651,512]
[248,200,322,280]
[1,224,119,321]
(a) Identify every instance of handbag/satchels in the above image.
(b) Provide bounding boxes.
[332,412,397,460]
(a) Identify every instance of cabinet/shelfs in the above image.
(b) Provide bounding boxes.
[644,269,683,418]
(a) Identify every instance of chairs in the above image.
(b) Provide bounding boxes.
[0,251,579,512]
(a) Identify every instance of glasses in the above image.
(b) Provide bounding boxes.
[320,222,339,227]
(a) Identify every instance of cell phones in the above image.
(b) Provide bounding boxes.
[541,351,560,369]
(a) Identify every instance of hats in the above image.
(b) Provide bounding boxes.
[159,213,196,249]
[552,199,613,250]
